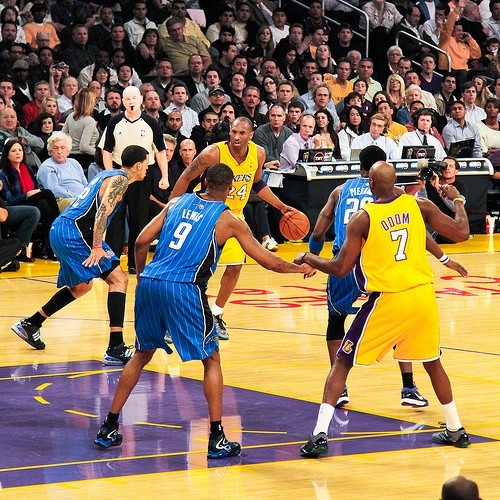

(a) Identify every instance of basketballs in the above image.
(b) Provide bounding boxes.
[279,210,310,240]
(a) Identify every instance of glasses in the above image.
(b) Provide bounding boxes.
[189,61,202,65]
[157,66,173,69]
[222,33,232,36]
[390,79,400,83]
[211,92,224,96]
[38,39,49,42]
[264,65,275,70]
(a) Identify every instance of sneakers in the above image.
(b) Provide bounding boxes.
[103,343,135,366]
[207,432,241,458]
[262,237,278,251]
[335,384,349,408]
[431,427,471,448]
[300,432,329,455]
[94,421,123,448]
[163,330,174,344]
[212,314,229,340]
[401,381,429,407]
[11,318,46,349]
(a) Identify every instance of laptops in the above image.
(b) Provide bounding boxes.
[299,148,332,162]
[350,148,363,161]
[450,137,476,158]
[401,146,435,158]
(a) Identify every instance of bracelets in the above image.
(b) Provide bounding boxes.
[92,246,102,249]
[453,198,466,205]
[438,253,449,264]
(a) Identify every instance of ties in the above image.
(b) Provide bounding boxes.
[141,22,147,30]
[423,135,428,145]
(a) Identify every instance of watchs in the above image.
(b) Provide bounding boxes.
[302,252,307,262]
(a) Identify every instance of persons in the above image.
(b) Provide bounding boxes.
[0,0,500,272]
[292,159,469,457]
[309,146,467,408]
[169,117,298,339]
[94,163,316,459]
[97,86,169,274]
[416,157,465,244]
[442,476,479,500]
[9,145,149,366]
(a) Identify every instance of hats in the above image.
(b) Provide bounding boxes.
[12,59,29,69]
[209,85,224,95]
[30,3,47,13]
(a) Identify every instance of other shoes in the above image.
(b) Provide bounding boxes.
[3,241,58,272]
[127,267,137,274]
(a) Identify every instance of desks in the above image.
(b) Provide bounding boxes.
[267,160,489,243]
